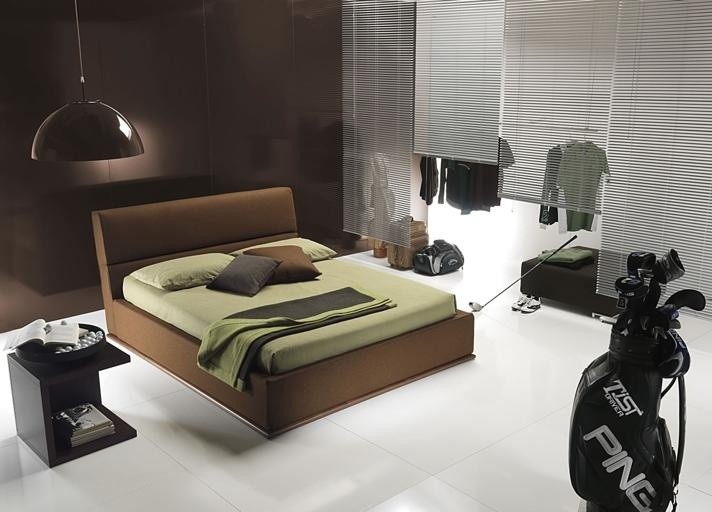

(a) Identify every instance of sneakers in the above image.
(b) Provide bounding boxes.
[521,296,541,313]
[512,295,532,310]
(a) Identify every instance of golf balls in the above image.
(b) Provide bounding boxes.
[52,330,102,355]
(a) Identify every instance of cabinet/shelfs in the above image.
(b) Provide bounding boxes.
[6,339,137,468]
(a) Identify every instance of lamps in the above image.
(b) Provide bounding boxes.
[24,0,146,160]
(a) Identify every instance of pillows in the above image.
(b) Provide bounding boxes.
[127,251,233,291]
[204,254,283,296]
[238,245,323,284]
[228,234,340,262]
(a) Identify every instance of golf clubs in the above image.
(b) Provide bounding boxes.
[469,235,579,311]
[613,248,705,336]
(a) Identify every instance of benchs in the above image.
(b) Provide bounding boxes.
[520,246,647,316]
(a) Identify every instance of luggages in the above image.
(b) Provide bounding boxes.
[388,216,429,270]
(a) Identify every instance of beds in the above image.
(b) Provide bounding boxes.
[87,184,475,438]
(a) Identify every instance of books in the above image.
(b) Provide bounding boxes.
[4,318,81,348]
[53,421,117,448]
[52,402,113,437]
